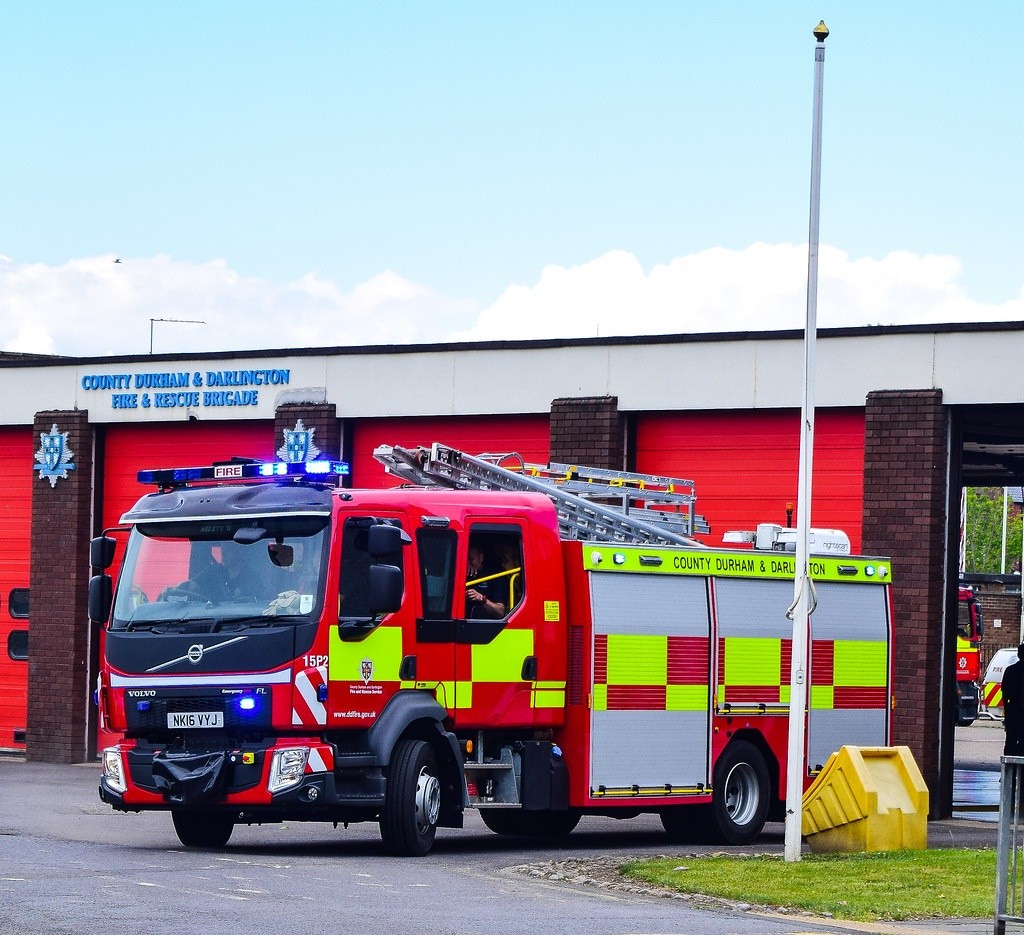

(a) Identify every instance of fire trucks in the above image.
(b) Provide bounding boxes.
[954,582,984,728]
[87,417,899,859]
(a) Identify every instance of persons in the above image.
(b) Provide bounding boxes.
[1001,644,1024,759]
[166,541,255,602]
[296,542,352,616]
[444,543,522,619]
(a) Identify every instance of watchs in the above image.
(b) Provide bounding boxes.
[479,594,487,605]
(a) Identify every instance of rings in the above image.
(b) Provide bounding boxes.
[476,597,478,599]
